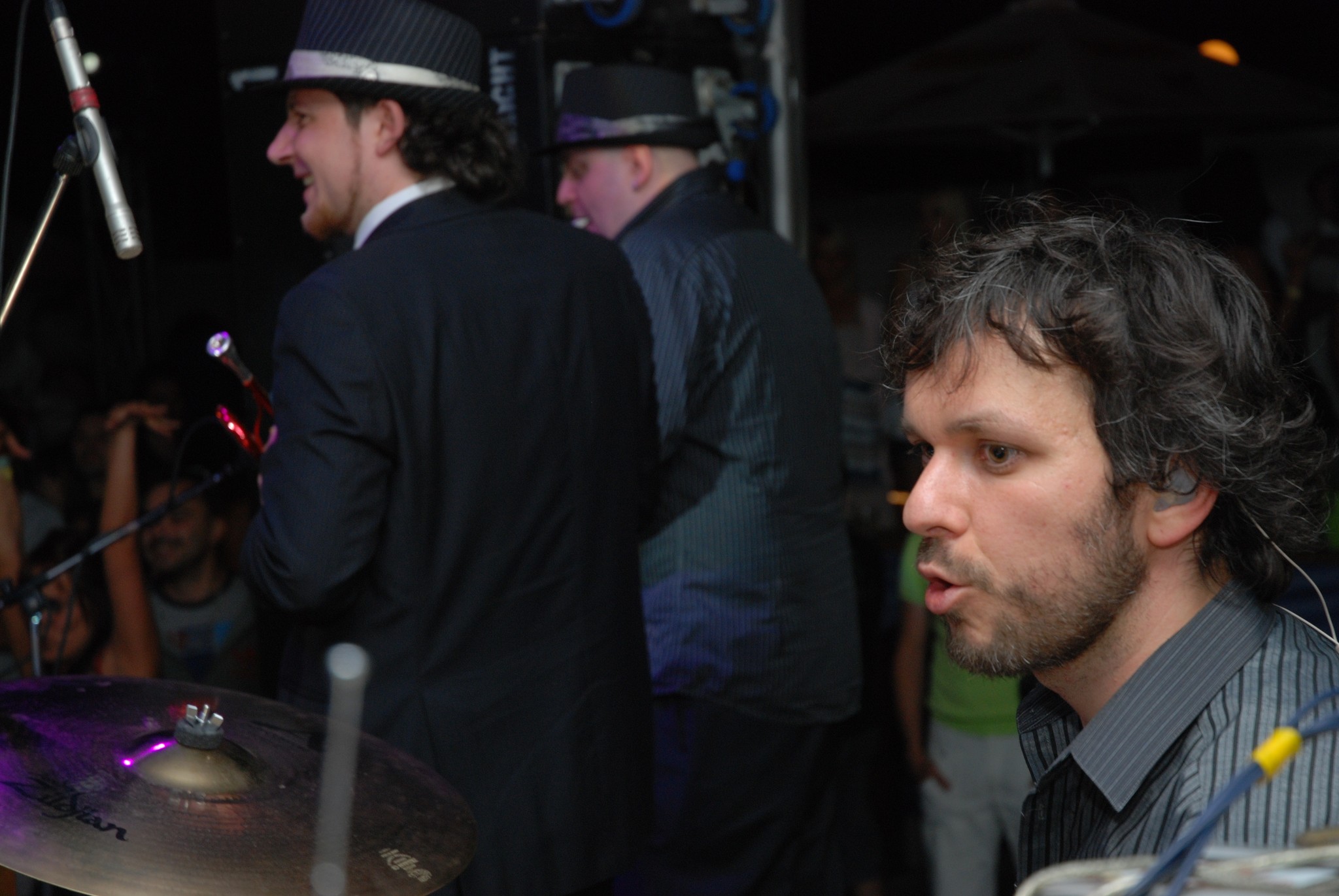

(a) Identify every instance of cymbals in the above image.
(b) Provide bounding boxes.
[0,674,476,896]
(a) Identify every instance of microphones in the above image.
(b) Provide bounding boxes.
[43,0,144,262]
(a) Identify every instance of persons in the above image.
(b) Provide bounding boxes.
[552,64,887,896]
[0,400,249,689]
[246,0,679,896]
[897,534,1035,896]
[868,202,1339,896]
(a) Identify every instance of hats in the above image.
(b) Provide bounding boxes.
[542,67,714,152]
[281,1,490,93]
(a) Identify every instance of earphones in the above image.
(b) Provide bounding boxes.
[1152,468,1199,512]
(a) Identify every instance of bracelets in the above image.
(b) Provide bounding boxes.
[107,414,132,435]
[0,456,14,482]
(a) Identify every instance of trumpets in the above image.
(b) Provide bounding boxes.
[207,332,278,457]
